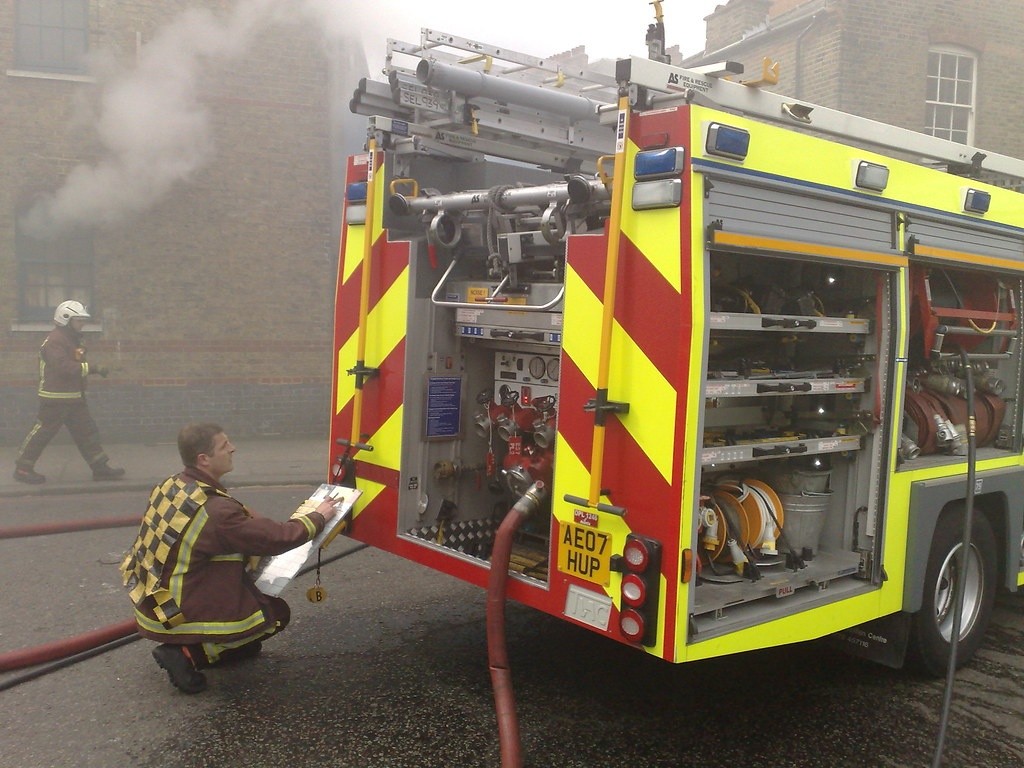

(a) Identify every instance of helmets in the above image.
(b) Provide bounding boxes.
[54,300,90,326]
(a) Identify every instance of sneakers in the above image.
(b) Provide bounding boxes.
[152,643,207,693]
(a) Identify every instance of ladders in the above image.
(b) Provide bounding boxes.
[386,26,621,107]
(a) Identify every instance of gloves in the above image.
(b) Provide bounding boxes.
[101,368,109,377]
[88,364,97,373]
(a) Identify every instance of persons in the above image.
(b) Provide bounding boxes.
[13,300,123,484]
[119,422,344,693]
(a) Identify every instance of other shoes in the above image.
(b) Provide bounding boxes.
[13,467,45,484]
[93,464,125,481]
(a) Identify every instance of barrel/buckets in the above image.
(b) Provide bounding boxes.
[779,490,833,556]
[777,464,832,494]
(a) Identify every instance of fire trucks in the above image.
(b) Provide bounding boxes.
[328,0,1024,680]
